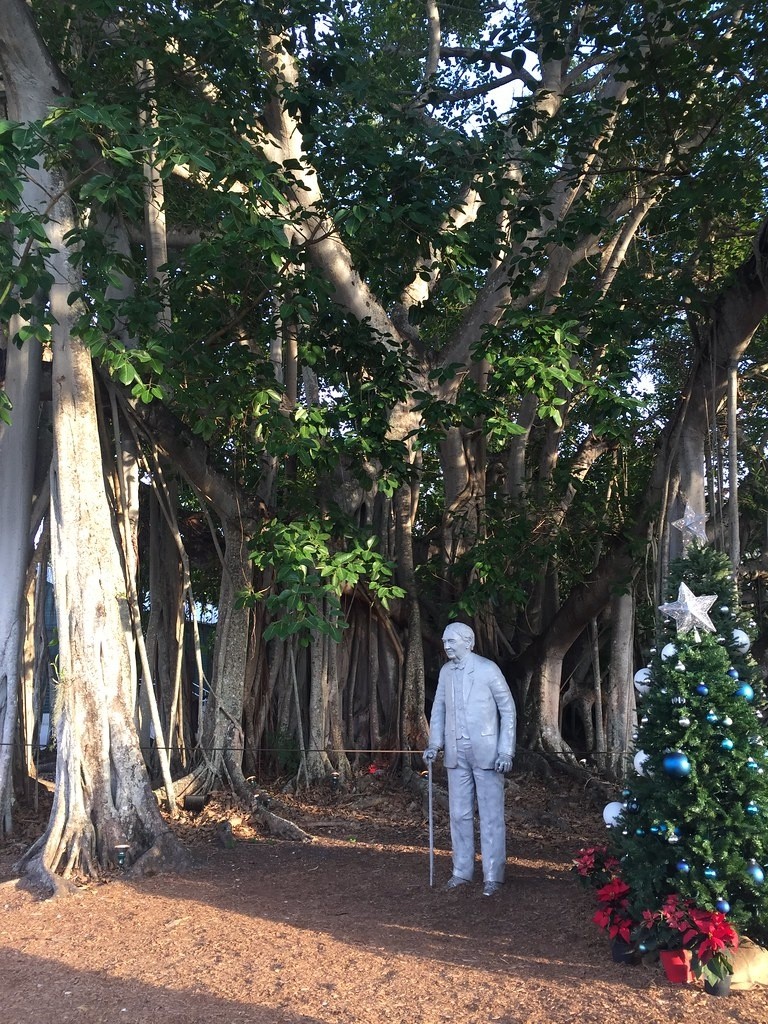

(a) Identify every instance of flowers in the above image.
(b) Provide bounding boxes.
[592,878,640,946]
[641,894,698,949]
[678,896,739,987]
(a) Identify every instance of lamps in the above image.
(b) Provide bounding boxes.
[184,795,205,813]
[115,844,130,869]
[332,772,340,789]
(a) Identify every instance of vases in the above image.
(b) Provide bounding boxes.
[659,949,694,982]
[705,976,731,996]
[612,942,636,966]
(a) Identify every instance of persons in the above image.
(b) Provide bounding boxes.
[421,622,517,895]
